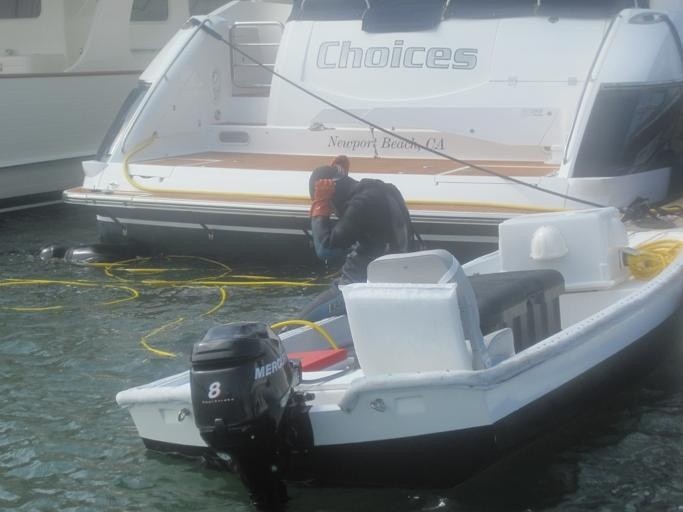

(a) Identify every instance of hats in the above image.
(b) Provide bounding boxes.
[309,165,344,198]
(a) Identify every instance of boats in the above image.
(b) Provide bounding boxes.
[0,1,240,213]
[62,0,683,255]
[114,224,683,489]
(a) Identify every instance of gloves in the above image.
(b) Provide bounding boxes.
[310,179,336,217]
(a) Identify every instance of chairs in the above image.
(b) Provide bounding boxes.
[340,251,516,373]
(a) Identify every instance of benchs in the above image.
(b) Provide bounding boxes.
[469,269,569,343]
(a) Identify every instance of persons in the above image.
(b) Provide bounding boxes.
[278,165,411,335]
